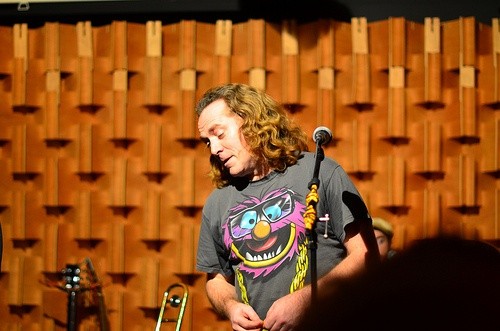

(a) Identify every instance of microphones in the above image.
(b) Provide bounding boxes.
[312,126,333,145]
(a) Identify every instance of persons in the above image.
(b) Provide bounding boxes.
[370,216,395,258]
[196,83,381,331]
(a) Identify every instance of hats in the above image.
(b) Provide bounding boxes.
[372,217,393,240]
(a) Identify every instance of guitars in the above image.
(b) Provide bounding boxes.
[63,264,81,331]
